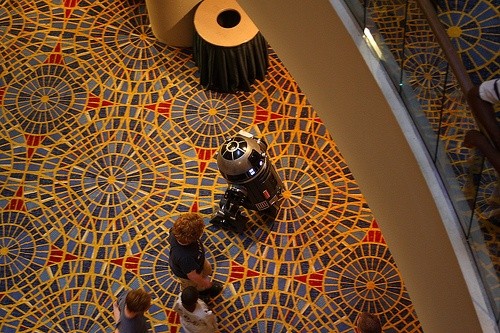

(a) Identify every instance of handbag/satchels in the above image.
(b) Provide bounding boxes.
[198,282,223,298]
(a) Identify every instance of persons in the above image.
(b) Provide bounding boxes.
[168,214,221,295]
[113,290,152,333]
[172,285,222,333]
[469,77,500,161]
[354,313,384,333]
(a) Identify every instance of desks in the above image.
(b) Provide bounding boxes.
[193,0,269,93]
